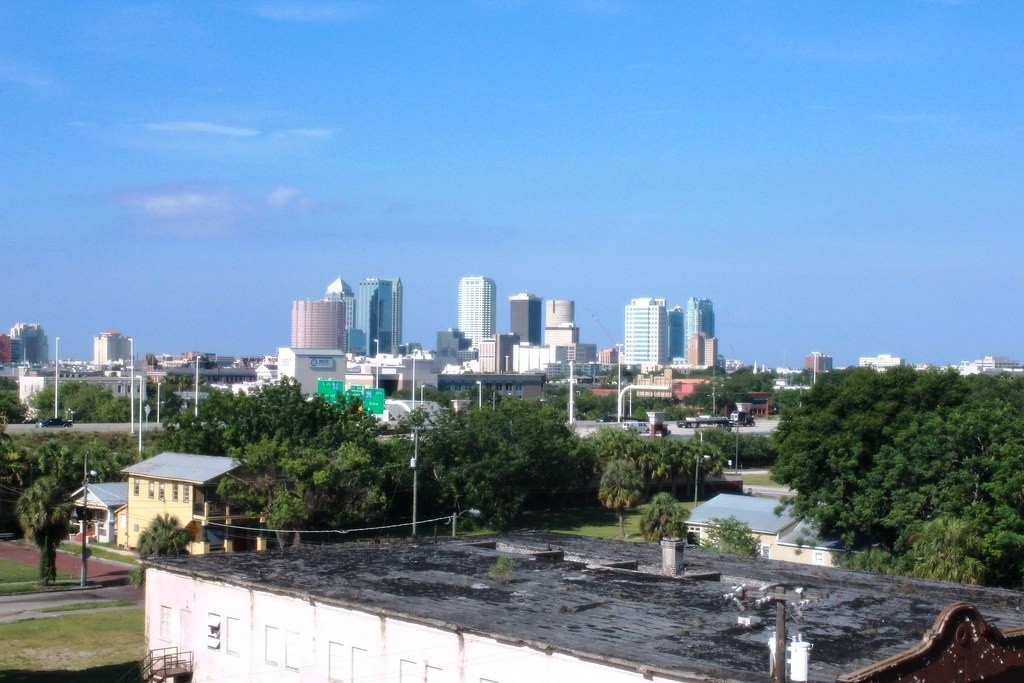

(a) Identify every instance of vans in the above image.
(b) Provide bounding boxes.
[623,422,651,433]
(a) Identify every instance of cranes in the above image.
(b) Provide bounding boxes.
[585,305,617,347]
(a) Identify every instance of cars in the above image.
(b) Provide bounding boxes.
[35,417,72,428]
[596,414,615,422]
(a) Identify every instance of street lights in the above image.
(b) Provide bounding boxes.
[411,349,419,411]
[410,426,418,537]
[373,339,379,389]
[80,469,99,587]
[157,381,162,425]
[128,337,135,435]
[136,374,143,454]
[452,507,482,538]
[195,355,202,416]
[475,380,482,410]
[54,337,61,418]
[615,343,624,398]
[567,360,575,426]
[420,385,426,405]
